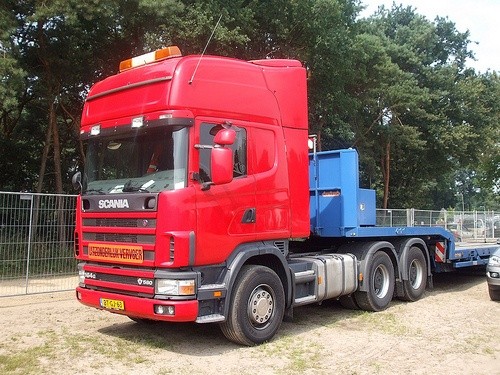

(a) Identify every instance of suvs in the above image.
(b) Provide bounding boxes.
[442,216,500,238]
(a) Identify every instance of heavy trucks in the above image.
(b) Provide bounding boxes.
[73,46,500,347]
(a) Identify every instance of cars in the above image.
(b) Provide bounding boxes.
[486,239,500,299]
